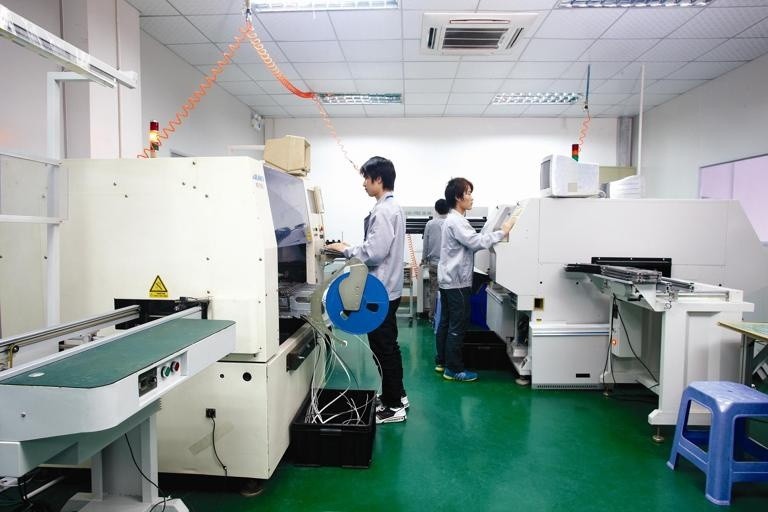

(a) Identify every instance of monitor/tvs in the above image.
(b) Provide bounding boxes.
[606,175,641,198]
[314,186,325,213]
[540,154,599,198]
[264,135,311,175]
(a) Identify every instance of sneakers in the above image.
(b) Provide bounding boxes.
[435,363,479,381]
[374,393,411,424]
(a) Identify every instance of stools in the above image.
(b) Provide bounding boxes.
[666,381,767,505]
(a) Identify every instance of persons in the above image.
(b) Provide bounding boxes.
[324,156,412,427]
[419,197,453,328]
[433,176,517,382]
